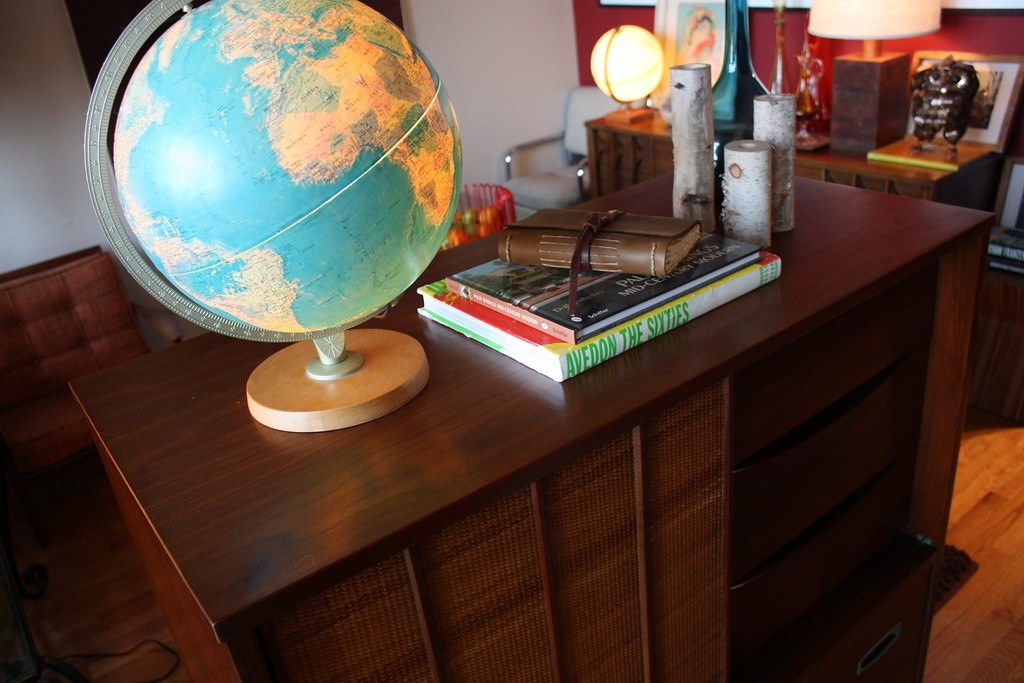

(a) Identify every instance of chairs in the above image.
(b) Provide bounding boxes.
[503,85,647,210]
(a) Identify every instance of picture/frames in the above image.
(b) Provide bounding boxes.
[906,50,1024,153]
[993,156,1024,232]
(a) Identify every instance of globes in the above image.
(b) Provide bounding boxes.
[79,1,467,436]
[590,24,665,125]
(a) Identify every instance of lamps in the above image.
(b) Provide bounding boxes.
[591,23,664,124]
[807,0,942,153]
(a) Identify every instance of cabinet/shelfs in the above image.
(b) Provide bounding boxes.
[64,174,1001,683]
[581,105,1003,209]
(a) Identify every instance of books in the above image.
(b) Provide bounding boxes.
[415,208,782,383]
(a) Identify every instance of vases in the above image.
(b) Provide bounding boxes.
[711,0,771,189]
